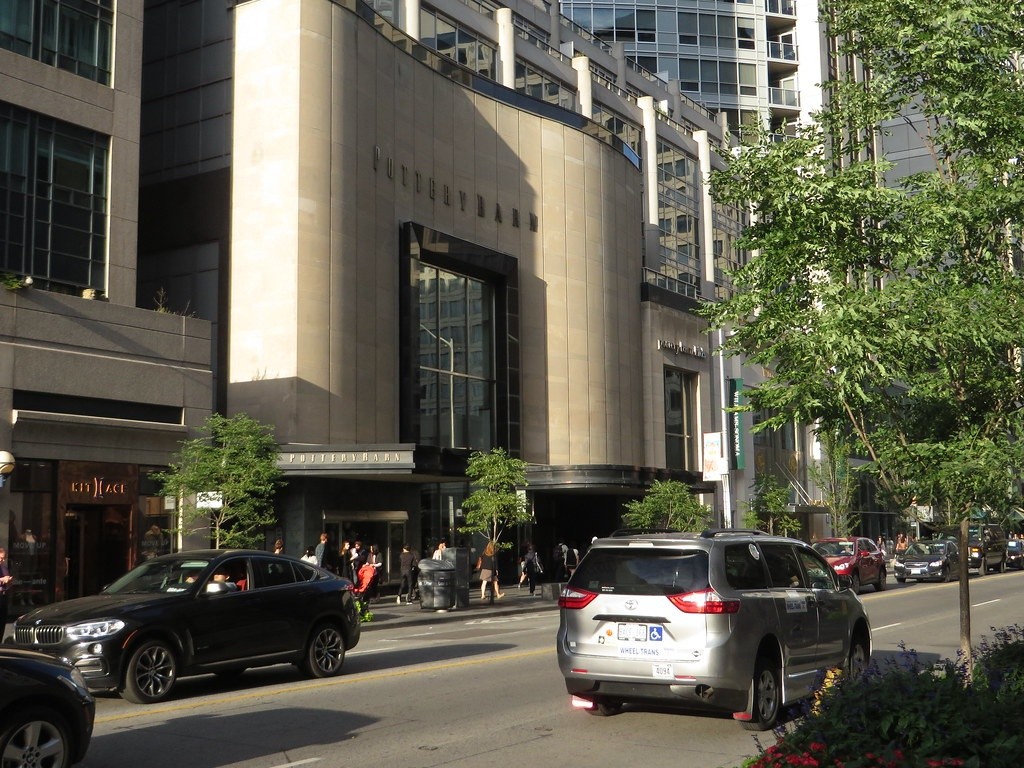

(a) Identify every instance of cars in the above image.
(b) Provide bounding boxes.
[1006,539,1024,570]
[0,647,95,768]
[810,537,887,595]
[12,548,363,704]
[894,539,960,583]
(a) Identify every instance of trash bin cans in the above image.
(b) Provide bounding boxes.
[417,568,455,608]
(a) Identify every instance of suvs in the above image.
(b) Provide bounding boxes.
[966,523,1008,577]
[557,527,873,731]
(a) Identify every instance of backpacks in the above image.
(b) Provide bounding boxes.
[525,554,537,575]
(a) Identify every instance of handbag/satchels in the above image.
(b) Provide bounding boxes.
[476,556,482,569]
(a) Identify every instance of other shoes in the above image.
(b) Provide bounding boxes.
[481,596,487,599]
[406,600,413,604]
[397,595,401,604]
[497,593,505,599]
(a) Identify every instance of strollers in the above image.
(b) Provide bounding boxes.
[352,562,377,624]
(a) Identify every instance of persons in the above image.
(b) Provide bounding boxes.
[269,539,284,573]
[0,546,13,645]
[1009,531,1024,539]
[896,534,908,554]
[301,533,384,588]
[479,542,504,599]
[186,564,237,592]
[877,536,893,551]
[558,542,579,575]
[841,545,853,554]
[432,542,446,560]
[397,544,417,605]
[517,538,540,596]
